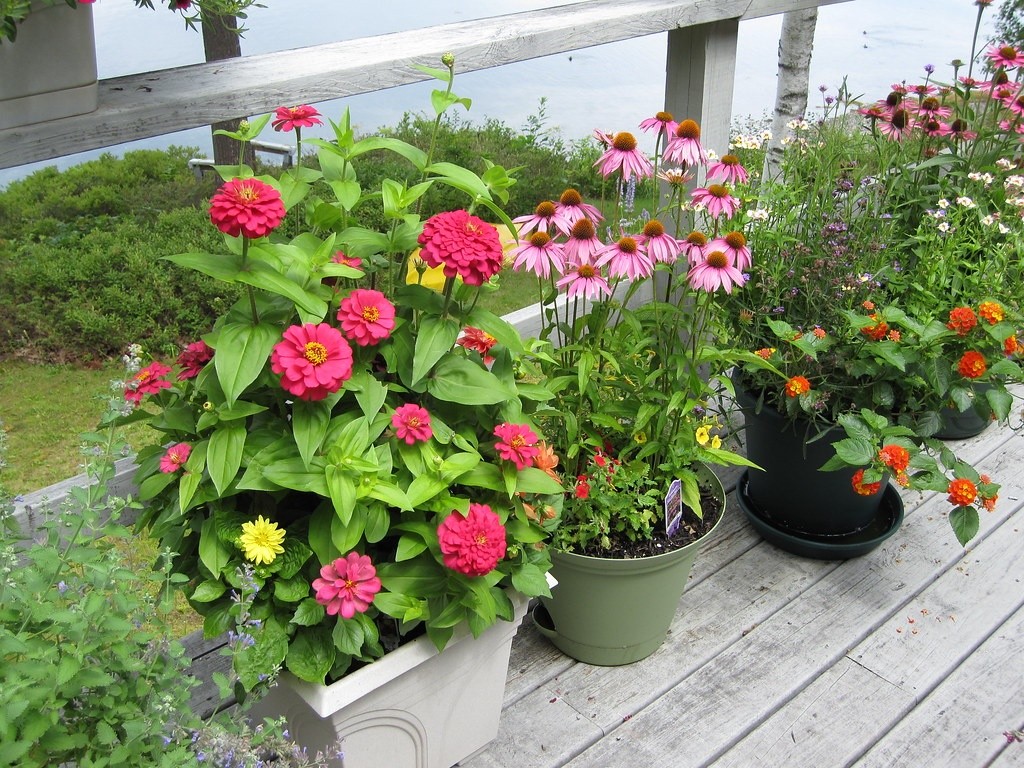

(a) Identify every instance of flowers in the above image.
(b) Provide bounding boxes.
[0,0,1024,768]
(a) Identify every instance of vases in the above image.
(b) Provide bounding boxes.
[916,348,1008,439]
[730,363,925,561]
[244,564,558,768]
[531,457,726,666]
